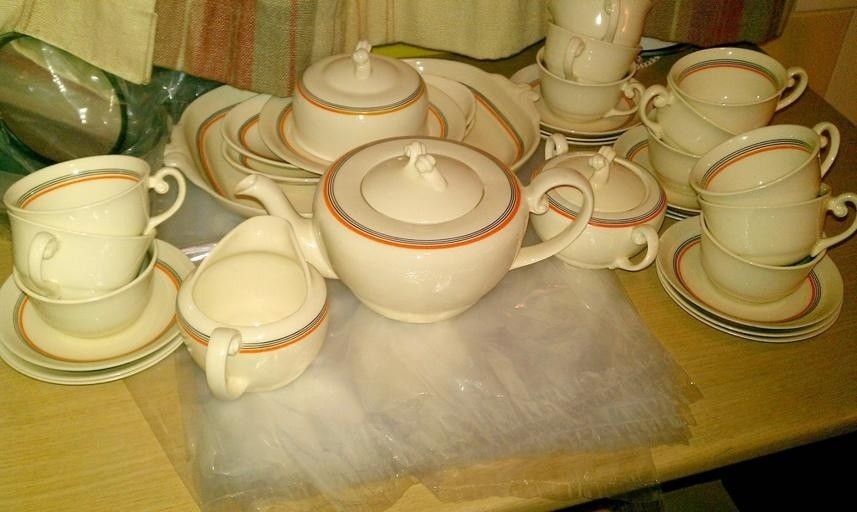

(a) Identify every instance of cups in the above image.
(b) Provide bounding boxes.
[533,0,856,305]
[0,153,187,341]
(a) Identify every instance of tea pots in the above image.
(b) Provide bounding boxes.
[236,132,596,326]
[172,213,329,403]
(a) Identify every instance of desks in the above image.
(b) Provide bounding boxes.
[0,79,856,512]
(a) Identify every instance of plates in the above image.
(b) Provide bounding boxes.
[162,41,542,217]
[654,213,846,344]
[1,241,199,387]
